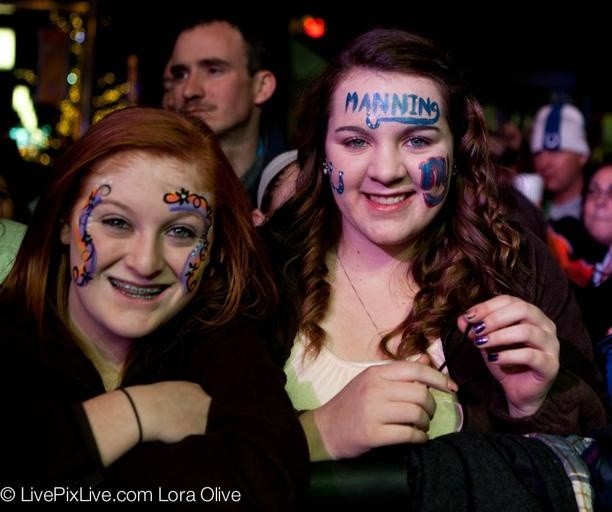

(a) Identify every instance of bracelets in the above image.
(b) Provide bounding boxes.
[122,387,144,446]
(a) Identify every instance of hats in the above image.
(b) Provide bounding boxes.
[529,102,592,159]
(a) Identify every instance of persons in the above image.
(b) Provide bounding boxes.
[0,105,309,512]
[251,146,298,225]
[172,14,285,200]
[0,61,170,285]
[228,25,612,512]
[486,102,612,319]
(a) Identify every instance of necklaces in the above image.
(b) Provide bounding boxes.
[334,246,396,354]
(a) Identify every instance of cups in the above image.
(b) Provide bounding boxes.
[509,173,545,210]
